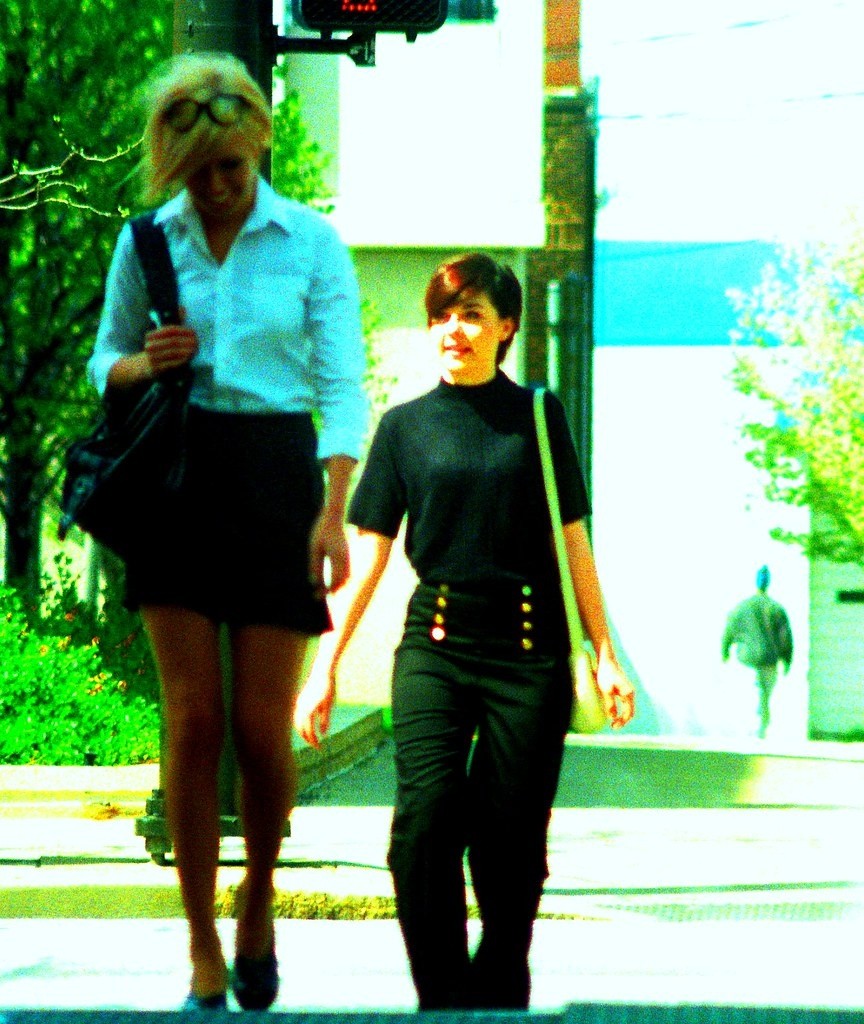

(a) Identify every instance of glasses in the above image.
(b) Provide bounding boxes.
[161,92,252,133]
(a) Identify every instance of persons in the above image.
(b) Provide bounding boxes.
[89,52,368,1011]
[293,252,634,1010]
[720,565,794,738]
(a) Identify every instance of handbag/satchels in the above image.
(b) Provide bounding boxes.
[53,212,196,559]
[574,651,604,731]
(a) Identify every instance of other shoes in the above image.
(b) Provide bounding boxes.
[230,906,280,1011]
[182,990,229,1010]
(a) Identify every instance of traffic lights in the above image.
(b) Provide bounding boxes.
[291,0,448,33]
